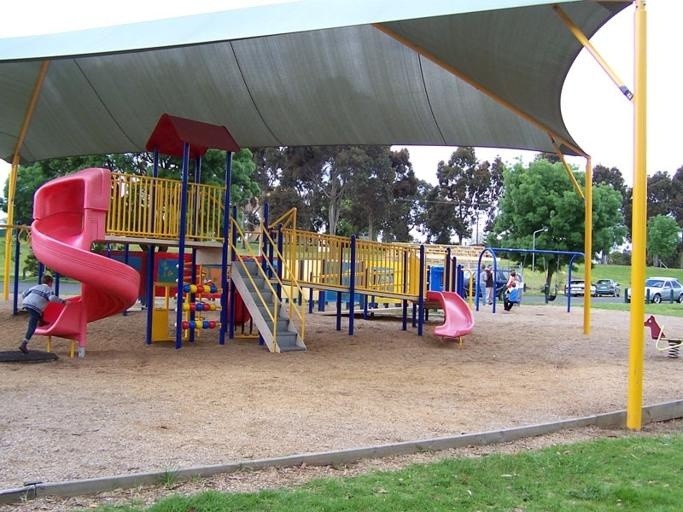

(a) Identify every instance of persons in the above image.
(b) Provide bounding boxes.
[502,283,519,311]
[506,272,519,288]
[246,198,262,244]
[473,264,486,306]
[239,200,254,237]
[18,275,66,354]
[484,264,493,304]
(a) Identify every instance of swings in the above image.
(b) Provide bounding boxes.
[543,253,560,301]
[504,252,527,303]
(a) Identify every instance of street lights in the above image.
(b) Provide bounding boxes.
[531,226,549,272]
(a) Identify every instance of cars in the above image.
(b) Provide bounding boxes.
[565,279,620,297]
[628,277,683,304]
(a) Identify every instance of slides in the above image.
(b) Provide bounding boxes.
[427,290,473,338]
[33,168,141,340]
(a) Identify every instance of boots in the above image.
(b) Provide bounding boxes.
[19,341,28,353]
